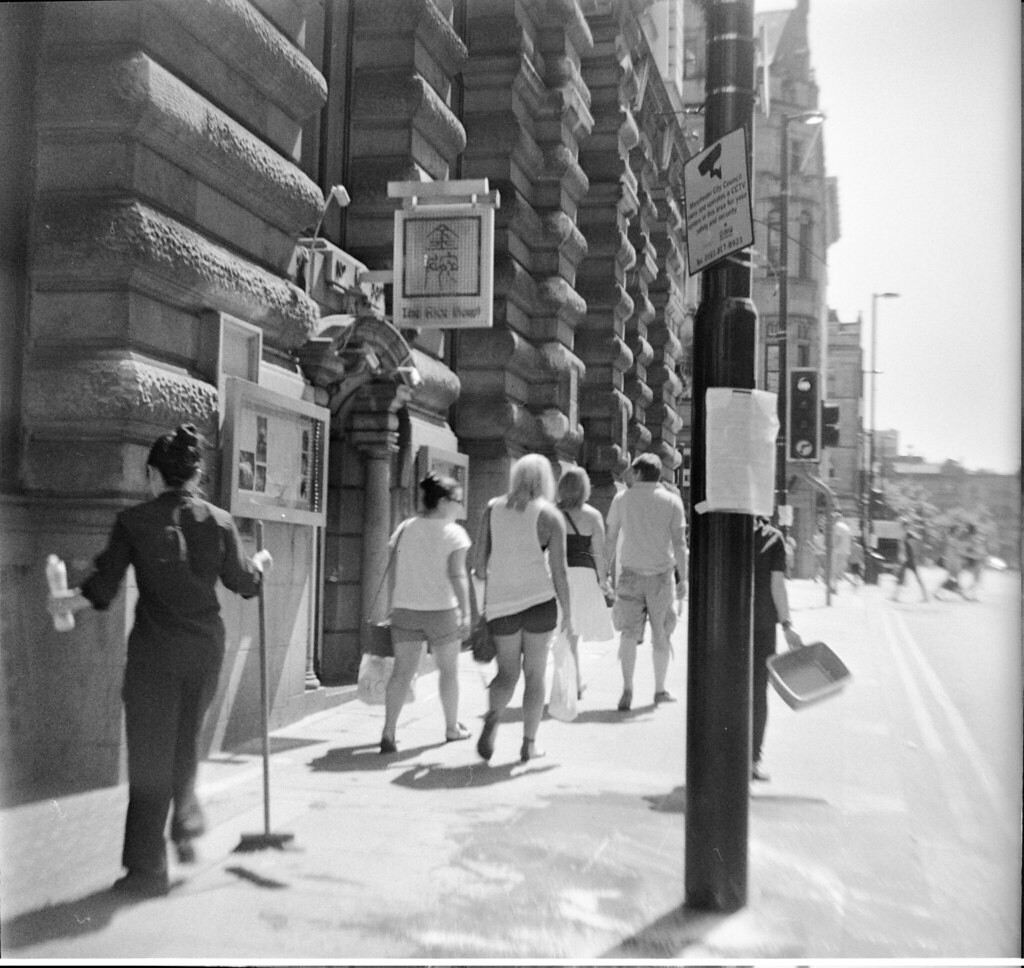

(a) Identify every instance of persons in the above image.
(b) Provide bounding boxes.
[473,451,573,761]
[783,512,986,607]
[606,453,687,712]
[47,424,275,902]
[378,471,474,754]
[753,517,807,782]
[541,464,610,723]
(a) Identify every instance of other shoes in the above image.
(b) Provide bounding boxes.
[445,722,472,741]
[618,690,633,710]
[114,868,168,897]
[521,737,545,759]
[654,691,677,707]
[753,762,770,779]
[380,731,397,752]
[477,711,500,760]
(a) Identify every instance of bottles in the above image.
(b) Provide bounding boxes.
[45,554,75,631]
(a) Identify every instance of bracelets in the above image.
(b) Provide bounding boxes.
[782,619,793,629]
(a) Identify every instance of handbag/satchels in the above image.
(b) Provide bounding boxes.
[547,628,592,721]
[356,620,417,704]
[472,616,496,662]
[597,571,615,607]
[943,577,958,590]
